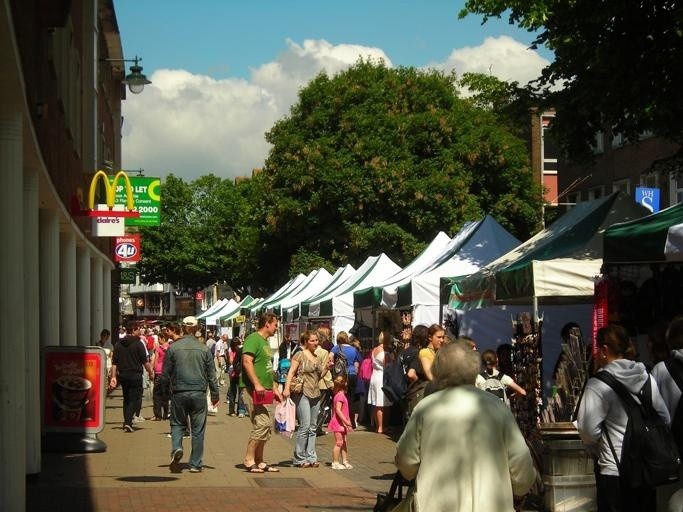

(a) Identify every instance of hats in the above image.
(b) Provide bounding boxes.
[183,316,197,326]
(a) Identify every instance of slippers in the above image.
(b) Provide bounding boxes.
[240,463,279,473]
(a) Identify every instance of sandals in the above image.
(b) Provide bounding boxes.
[295,462,319,468]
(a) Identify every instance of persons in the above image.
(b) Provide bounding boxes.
[650,315,683,446]
[316,325,454,470]
[574,321,683,512]
[475,319,591,407]
[158,313,221,474]
[97,326,127,376]
[281,329,334,471]
[108,320,154,433]
[237,311,282,473]
[132,321,182,422]
[392,341,536,511]
[273,334,306,433]
[195,331,247,417]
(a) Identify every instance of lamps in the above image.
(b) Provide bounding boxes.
[99,56,152,96]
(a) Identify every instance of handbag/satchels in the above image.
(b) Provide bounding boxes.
[275,398,296,440]
[373,494,406,512]
[229,365,239,378]
[289,379,303,394]
[360,359,372,380]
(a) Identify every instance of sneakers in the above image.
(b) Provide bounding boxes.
[134,414,171,421]
[124,425,133,431]
[167,434,190,439]
[231,413,245,418]
[170,449,183,466]
[332,463,353,469]
[190,466,205,473]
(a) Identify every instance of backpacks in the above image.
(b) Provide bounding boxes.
[479,371,505,404]
[330,343,347,381]
[382,349,419,402]
[591,370,682,491]
